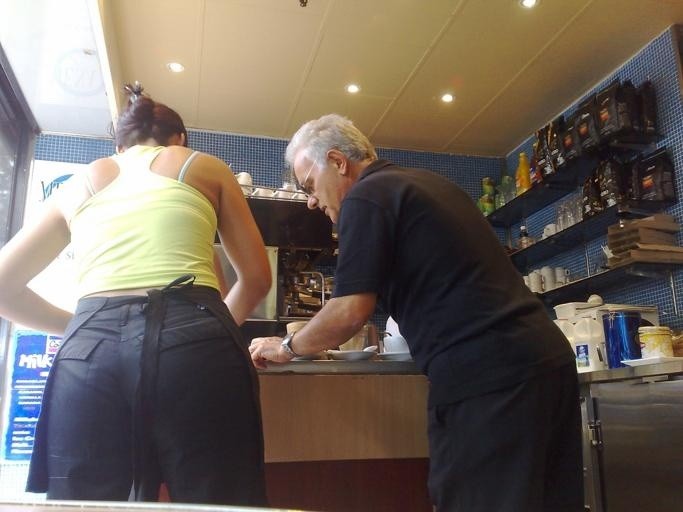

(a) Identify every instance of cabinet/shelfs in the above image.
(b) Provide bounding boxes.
[479,122,681,312]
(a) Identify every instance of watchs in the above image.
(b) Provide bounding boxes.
[281,330,303,358]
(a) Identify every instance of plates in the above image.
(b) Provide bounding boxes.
[379,352,412,363]
[619,356,682,366]
[328,351,377,361]
[292,352,321,361]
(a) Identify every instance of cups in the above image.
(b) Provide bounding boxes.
[381,335,407,352]
[232,172,309,202]
[554,183,584,232]
[285,320,307,336]
[601,311,654,368]
[543,223,556,237]
[523,265,570,295]
[340,333,364,350]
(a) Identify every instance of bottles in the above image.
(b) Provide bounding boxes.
[528,143,543,185]
[495,175,516,210]
[519,225,528,248]
[515,152,532,195]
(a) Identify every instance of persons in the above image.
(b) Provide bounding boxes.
[0,80,272,506]
[247,114,588,512]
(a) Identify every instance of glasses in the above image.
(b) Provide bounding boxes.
[294,147,338,196]
